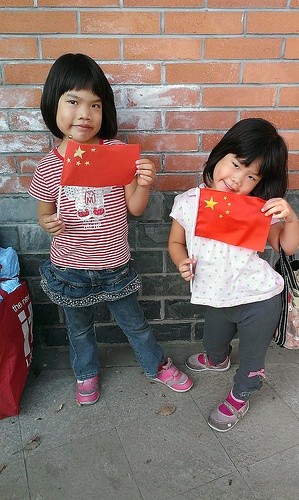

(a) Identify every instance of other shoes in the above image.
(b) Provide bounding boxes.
[208,387,250,432]
[184,352,232,372]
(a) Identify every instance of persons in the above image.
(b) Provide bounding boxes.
[167,117,299,434]
[28,53,194,405]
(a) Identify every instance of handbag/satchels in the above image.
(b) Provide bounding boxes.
[273,243,299,350]
[0,263,34,419]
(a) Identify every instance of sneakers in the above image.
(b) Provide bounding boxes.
[151,358,194,392]
[77,375,99,405]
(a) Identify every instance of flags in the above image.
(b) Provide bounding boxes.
[60,139,141,187]
[195,187,273,252]
